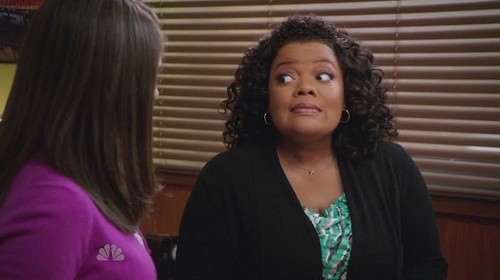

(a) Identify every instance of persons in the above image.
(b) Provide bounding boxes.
[1,0,164,280]
[177,17,448,280]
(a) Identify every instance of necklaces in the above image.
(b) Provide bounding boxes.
[284,153,329,174]
